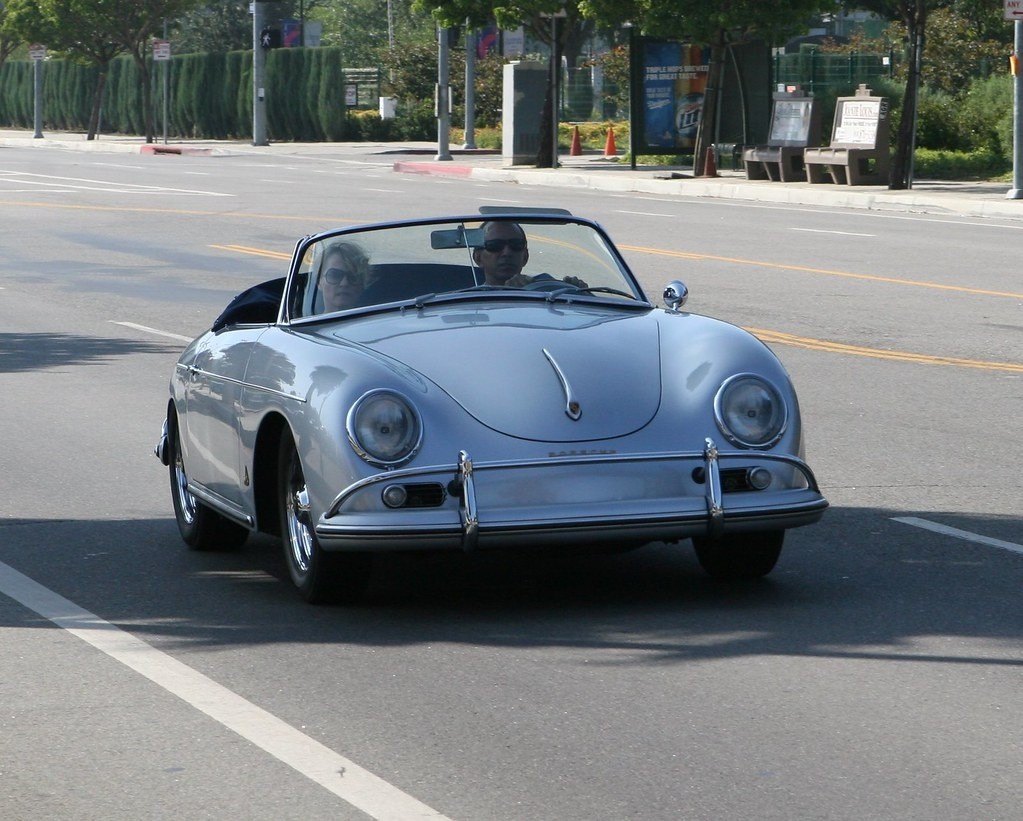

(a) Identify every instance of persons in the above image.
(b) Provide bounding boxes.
[318,244,373,312]
[473,222,590,292]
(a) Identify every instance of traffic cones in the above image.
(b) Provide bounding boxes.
[603,126,618,155]
[570,126,583,156]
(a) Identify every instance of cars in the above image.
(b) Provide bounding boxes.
[152,206,829,602]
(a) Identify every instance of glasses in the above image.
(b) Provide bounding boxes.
[477,239,527,253]
[323,268,364,286]
